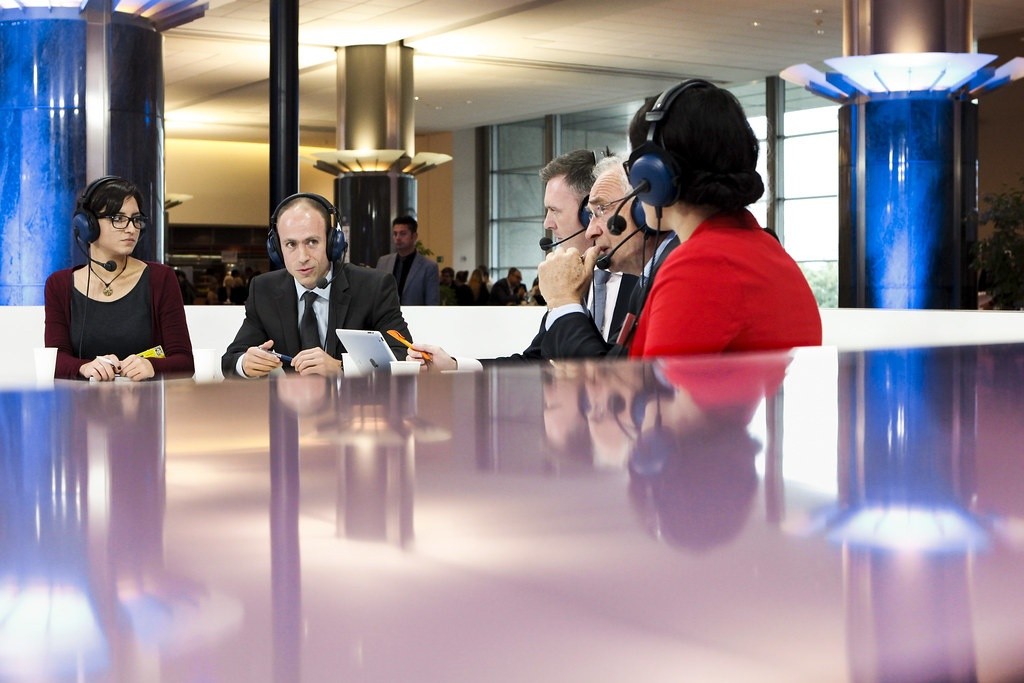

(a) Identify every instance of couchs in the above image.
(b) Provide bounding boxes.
[0,306,1024,384]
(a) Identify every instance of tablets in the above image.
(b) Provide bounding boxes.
[335,329,398,376]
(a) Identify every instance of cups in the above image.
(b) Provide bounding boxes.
[29,347,58,391]
[388,361,421,375]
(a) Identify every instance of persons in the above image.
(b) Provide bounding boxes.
[538,156,681,360]
[175,267,261,305]
[377,217,441,306]
[407,149,639,371]
[623,78,822,358]
[276,374,332,417]
[543,352,796,551]
[45,175,196,381]
[440,264,546,306]
[220,192,413,379]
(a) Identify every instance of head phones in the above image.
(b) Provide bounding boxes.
[622,160,665,236]
[73,175,123,244]
[578,150,607,227]
[627,78,715,208]
[266,193,347,267]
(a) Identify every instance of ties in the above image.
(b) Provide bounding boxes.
[297,291,322,351]
[586,267,610,334]
[394,257,407,288]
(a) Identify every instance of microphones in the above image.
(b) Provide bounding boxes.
[607,179,650,235]
[316,242,348,289]
[72,226,117,272]
[594,223,646,270]
[539,228,587,250]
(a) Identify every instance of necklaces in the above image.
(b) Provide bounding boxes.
[90,256,127,296]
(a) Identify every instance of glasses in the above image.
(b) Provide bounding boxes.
[513,274,523,281]
[95,212,149,229]
[588,195,630,220]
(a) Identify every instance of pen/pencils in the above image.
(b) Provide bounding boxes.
[96,355,114,365]
[268,351,292,361]
[550,359,558,365]
[387,330,432,362]
